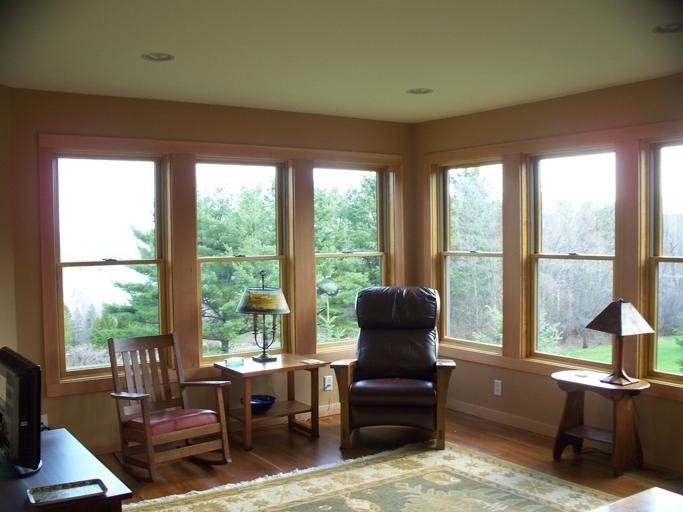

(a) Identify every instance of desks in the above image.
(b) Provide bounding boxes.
[550,369,651,481]
[0,421,132,512]
[212,351,332,452]
[589,486,682,511]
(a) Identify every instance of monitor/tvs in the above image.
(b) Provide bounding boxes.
[0,346,43,477]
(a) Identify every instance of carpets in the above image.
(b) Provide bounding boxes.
[118,433,624,512]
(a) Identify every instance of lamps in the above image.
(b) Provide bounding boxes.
[584,294,656,387]
[234,271,291,363]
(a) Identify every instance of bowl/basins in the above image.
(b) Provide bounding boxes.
[241,394,276,415]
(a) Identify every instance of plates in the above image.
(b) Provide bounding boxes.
[27,478,107,506]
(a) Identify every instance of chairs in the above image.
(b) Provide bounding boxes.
[330,285,457,458]
[105,330,233,487]
